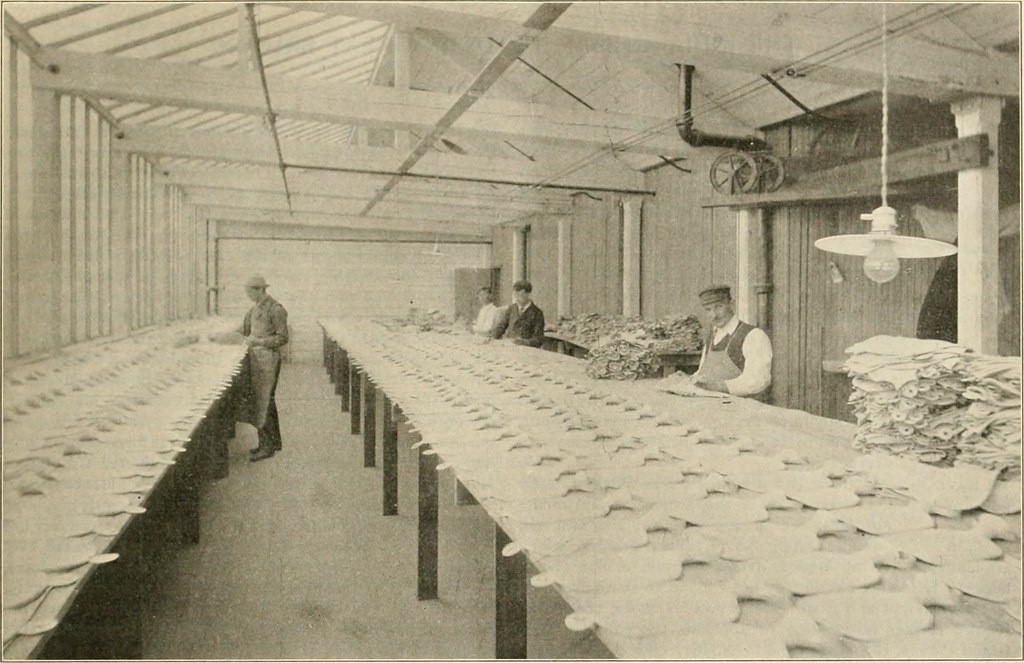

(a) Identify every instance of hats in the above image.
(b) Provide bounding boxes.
[699,285,730,305]
[242,273,270,288]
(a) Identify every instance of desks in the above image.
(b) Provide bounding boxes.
[3,313,1022,659]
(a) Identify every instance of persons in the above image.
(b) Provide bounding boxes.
[472,287,500,337]
[210,274,289,461]
[668,283,773,405]
[483,279,545,348]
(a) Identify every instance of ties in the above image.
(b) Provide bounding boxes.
[519,307,524,316]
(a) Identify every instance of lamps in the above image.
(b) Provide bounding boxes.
[814,3,960,284]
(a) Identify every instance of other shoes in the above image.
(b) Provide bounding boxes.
[251,443,282,454]
[249,447,275,461]
